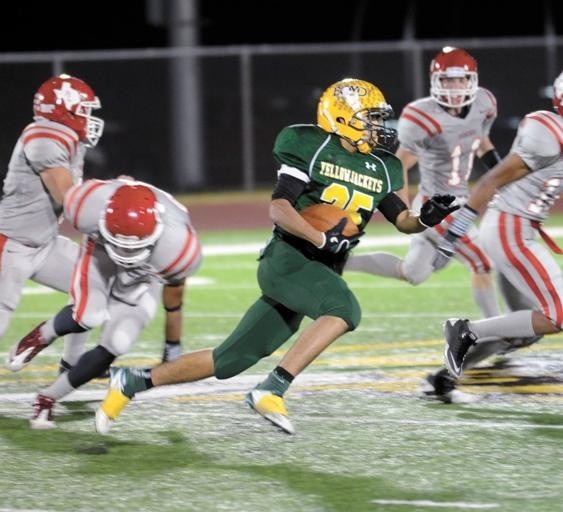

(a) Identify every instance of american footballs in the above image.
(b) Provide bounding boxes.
[298,205,359,238]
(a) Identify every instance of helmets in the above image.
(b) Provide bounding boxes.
[316,76,397,157]
[100,182,165,270]
[31,72,107,150]
[429,45,481,109]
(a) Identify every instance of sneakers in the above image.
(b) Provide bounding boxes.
[422,365,467,404]
[94,363,151,439]
[28,391,59,431]
[442,315,477,380]
[242,383,298,438]
[6,318,57,372]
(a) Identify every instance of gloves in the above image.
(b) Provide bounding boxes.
[418,194,461,229]
[317,232,361,258]
[427,233,459,274]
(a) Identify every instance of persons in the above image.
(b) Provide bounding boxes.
[3,175,200,430]
[0,74,104,376]
[342,45,513,366]
[95,77,464,433]
[416,70,562,405]
